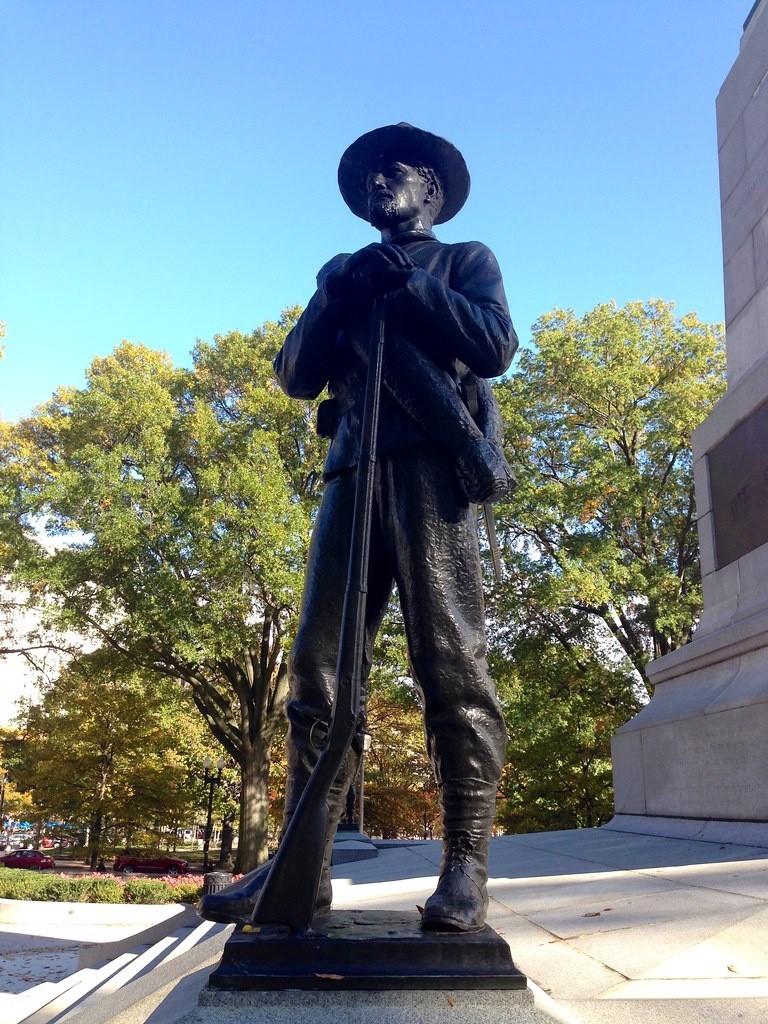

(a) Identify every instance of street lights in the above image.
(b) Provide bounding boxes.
[201,754,224,873]
[358,728,374,835]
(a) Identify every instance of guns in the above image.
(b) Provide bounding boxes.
[250,278,386,934]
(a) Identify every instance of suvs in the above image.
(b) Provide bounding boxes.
[112,848,190,874]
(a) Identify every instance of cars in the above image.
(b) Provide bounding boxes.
[0,834,79,852]
[0,849,55,870]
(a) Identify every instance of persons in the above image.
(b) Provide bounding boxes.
[206,125,519,931]
[43,836,51,848]
